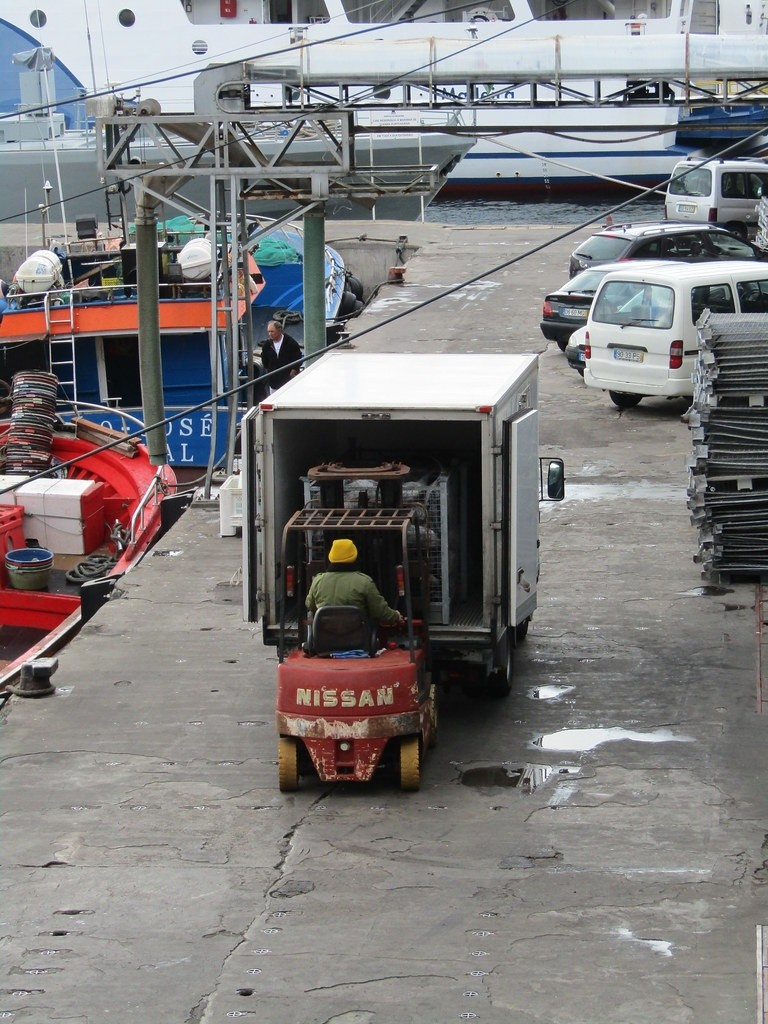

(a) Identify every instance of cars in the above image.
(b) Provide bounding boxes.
[566,285,673,376]
[538,260,689,351]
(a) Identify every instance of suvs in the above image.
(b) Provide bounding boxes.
[662,156,768,241]
[569,220,768,281]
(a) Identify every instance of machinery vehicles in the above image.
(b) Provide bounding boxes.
[274,506,440,796]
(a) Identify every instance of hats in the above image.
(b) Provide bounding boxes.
[328,540,358,563]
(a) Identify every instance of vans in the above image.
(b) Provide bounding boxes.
[584,261,768,409]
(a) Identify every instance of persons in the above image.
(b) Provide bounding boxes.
[306,539,401,634]
[261,321,303,394]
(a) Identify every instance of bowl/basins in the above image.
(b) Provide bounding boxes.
[5,548,54,590]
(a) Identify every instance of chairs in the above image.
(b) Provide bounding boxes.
[302,605,380,659]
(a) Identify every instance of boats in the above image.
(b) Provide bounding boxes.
[0,1,768,198]
[2,212,348,535]
[0,46,478,221]
[0,399,178,698]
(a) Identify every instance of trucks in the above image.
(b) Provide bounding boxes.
[239,350,567,695]
